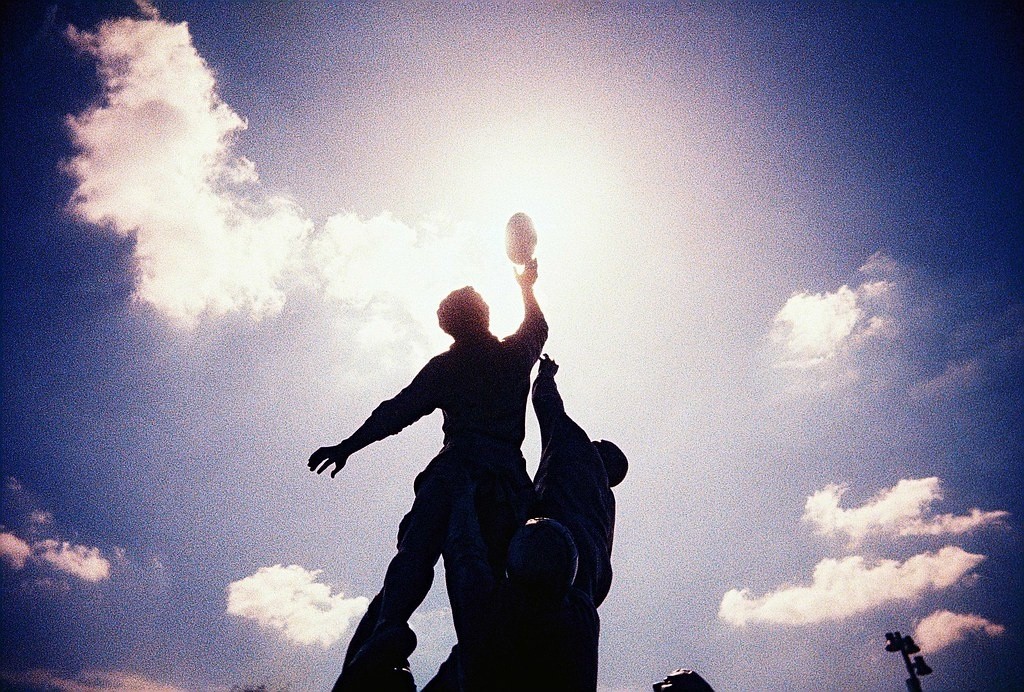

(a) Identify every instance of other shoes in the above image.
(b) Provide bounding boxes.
[341,623,417,679]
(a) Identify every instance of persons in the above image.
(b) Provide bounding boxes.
[308,256,628,692]
[653,669,715,692]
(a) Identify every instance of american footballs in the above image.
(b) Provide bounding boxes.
[504,211,538,264]
